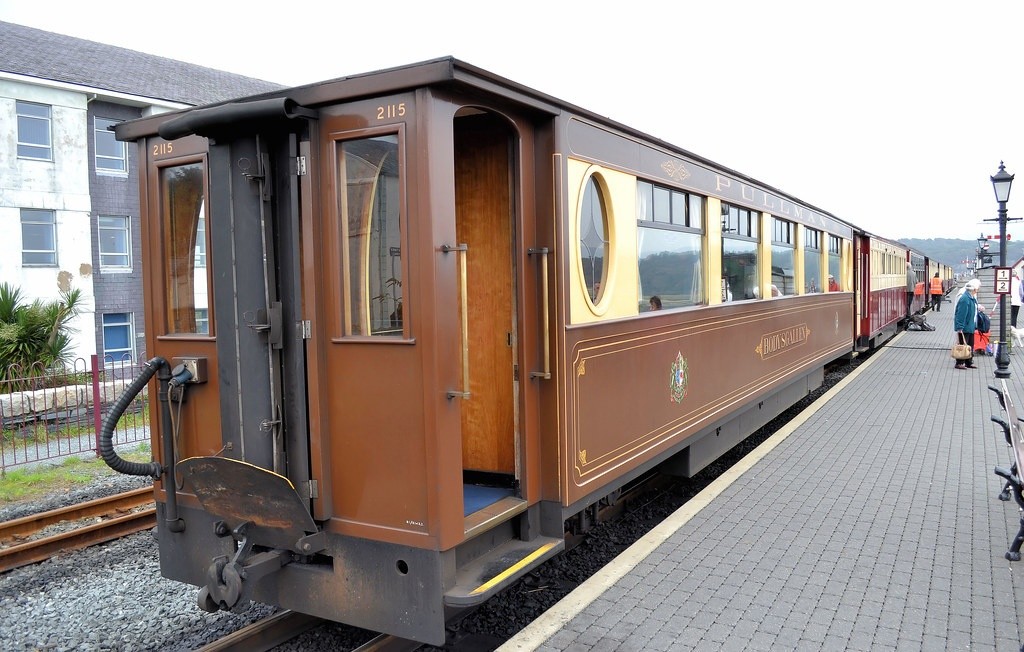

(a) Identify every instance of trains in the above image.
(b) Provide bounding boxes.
[98,54,955,647]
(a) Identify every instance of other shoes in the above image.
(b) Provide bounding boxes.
[955,364,967,369]
[966,363,977,368]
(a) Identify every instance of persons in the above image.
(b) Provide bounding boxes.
[906,262,918,316]
[951,278,991,369]
[771,285,781,298]
[1009,270,1023,330]
[808,277,817,293]
[592,280,602,299]
[828,274,841,293]
[928,272,942,312]
[648,295,662,310]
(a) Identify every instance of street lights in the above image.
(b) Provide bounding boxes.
[982,160,1024,378]
[977,233,989,267]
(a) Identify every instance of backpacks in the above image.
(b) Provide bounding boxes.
[976,310,991,332]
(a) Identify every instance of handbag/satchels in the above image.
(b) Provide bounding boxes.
[951,331,972,360]
[974,330,991,351]
